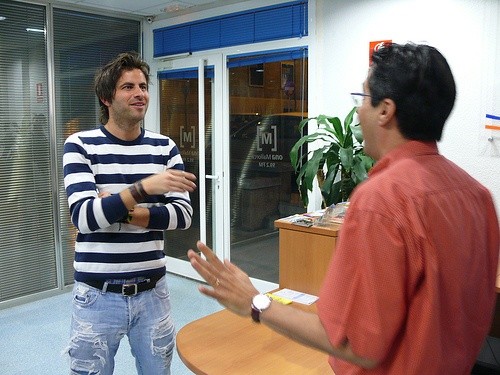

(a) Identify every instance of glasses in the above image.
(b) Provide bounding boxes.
[348,90,391,109]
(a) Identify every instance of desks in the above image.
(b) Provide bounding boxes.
[274,210,344,295]
[176,290,335,375]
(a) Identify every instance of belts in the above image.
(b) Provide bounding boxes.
[84,277,157,297]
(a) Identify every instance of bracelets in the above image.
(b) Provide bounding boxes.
[128,184,145,203]
[136,179,149,198]
[115,207,134,231]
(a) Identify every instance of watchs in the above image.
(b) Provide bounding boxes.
[250,293,273,322]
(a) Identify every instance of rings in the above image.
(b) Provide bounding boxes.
[211,278,220,287]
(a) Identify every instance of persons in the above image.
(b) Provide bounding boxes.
[62,50,196,375]
[188,41,500,375]
